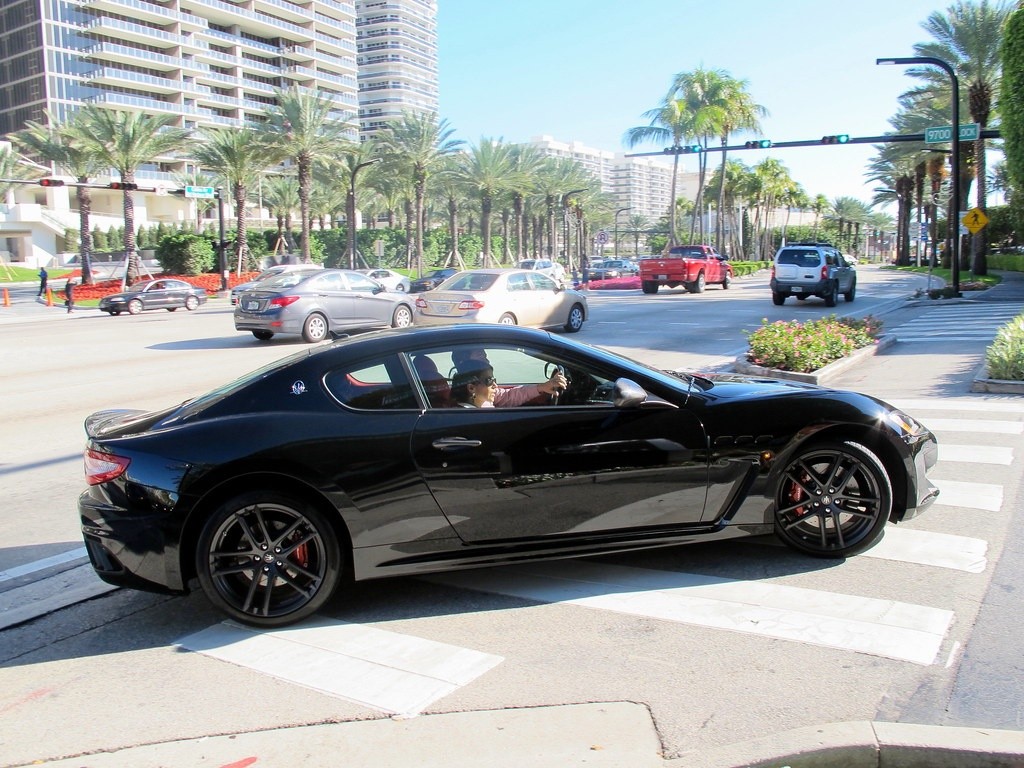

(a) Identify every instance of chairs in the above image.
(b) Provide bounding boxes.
[421,372,455,407]
[413,355,437,373]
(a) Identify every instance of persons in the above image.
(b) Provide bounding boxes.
[66,278,77,313]
[36,267,47,296]
[448,359,498,408]
[451,348,567,408]
[571,253,590,290]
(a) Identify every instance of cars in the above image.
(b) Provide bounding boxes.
[553,263,566,280]
[234,269,416,343]
[78,325,943,622]
[412,268,458,292]
[99,279,207,316]
[586,255,640,279]
[841,254,859,266]
[415,269,589,332]
[357,269,411,293]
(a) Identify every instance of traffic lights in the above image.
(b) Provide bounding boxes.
[664,145,701,154]
[745,140,770,149]
[110,182,137,191]
[822,135,849,145]
[39,179,64,187]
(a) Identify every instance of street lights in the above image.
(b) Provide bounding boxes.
[615,207,638,260]
[349,157,386,270]
[875,56,962,296]
[562,188,588,257]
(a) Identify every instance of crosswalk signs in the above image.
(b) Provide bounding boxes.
[962,208,990,237]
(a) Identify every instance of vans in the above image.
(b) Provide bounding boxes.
[514,259,555,281]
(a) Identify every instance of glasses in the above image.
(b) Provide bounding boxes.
[475,377,498,387]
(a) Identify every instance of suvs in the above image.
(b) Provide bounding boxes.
[770,241,856,308]
[230,264,325,306]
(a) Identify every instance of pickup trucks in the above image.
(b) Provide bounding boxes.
[640,245,735,294]
